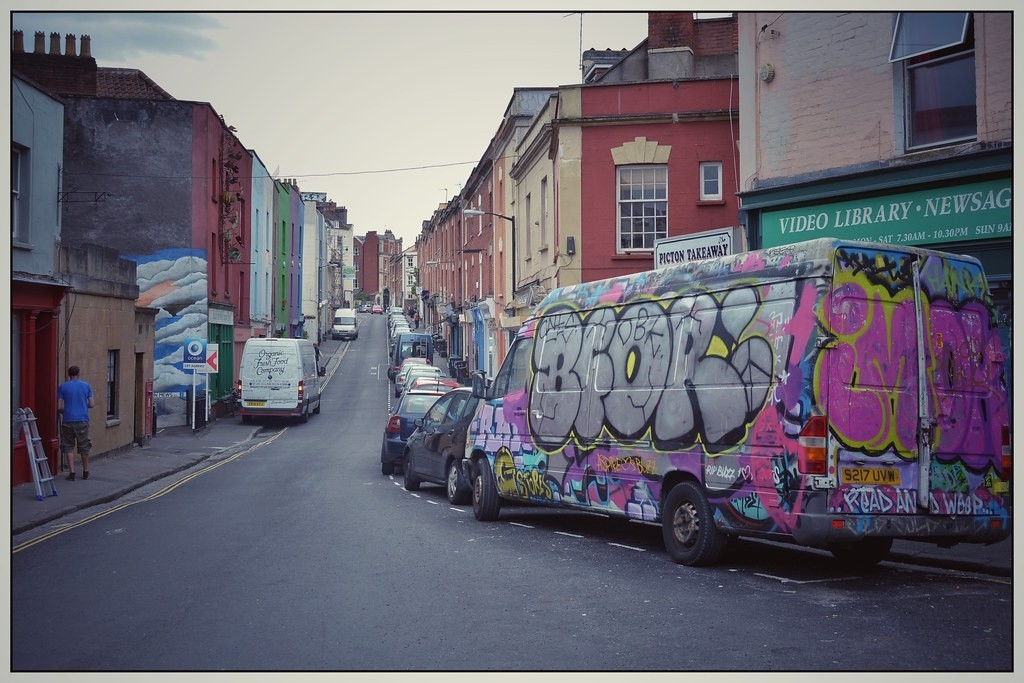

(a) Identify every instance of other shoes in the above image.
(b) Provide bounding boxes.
[65,472,75,481]
[82,471,92,480]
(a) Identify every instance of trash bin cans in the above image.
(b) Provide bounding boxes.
[153,402,157,437]
[434,336,446,354]
[450,357,467,383]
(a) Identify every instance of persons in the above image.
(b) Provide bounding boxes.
[407,304,419,328]
[57,366,94,480]
[313,343,324,374]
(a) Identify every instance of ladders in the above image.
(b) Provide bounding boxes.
[12,408,58,501]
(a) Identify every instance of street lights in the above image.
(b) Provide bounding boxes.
[463,209,516,344]
[343,289,354,308]
[317,261,339,347]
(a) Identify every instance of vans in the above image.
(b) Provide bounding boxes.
[235,336,327,423]
[459,237,1011,571]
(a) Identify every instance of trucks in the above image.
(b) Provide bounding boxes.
[330,307,359,340]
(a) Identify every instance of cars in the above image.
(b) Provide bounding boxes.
[385,305,460,397]
[402,386,480,506]
[356,304,372,313]
[370,305,383,315]
[380,389,465,475]
